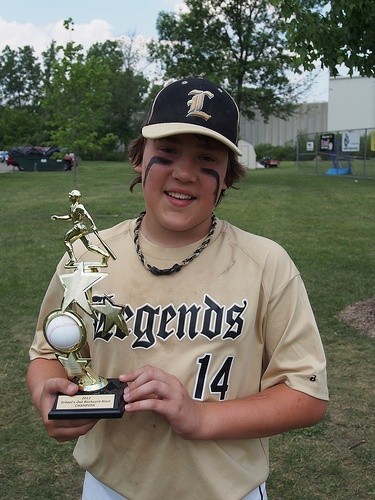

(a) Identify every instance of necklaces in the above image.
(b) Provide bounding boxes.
[133,209,218,274]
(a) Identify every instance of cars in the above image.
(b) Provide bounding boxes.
[259,156,279,168]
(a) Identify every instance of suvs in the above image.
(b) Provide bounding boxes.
[4,144,76,171]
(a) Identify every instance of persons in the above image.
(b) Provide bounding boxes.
[27,77,331,500]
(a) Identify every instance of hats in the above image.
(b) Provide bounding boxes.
[141,77,243,157]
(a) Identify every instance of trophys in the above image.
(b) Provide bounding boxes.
[42,188,132,421]
